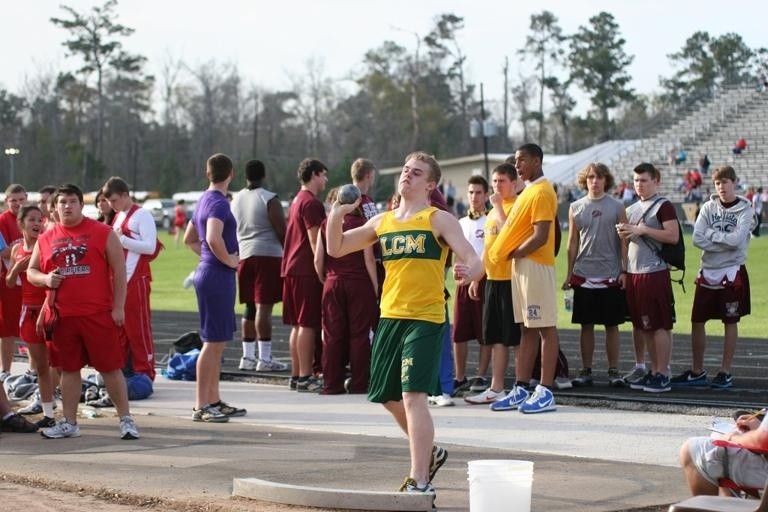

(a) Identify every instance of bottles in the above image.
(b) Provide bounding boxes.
[81,409,96,420]
[563,289,573,312]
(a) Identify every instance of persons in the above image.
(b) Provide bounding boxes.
[313,156,528,408]
[487,142,559,414]
[174,199,187,241]
[324,151,486,500]
[4,176,164,435]
[670,164,756,387]
[615,161,681,393]
[610,134,767,239]
[182,151,249,423]
[279,157,327,392]
[230,159,289,373]
[24,182,141,440]
[560,162,631,388]
[678,411,768,497]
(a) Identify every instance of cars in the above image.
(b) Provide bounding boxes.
[0,191,290,229]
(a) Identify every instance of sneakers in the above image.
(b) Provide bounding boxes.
[43,418,81,438]
[34,416,56,428]
[210,400,247,417]
[238,356,258,371]
[608,368,625,388]
[191,404,230,423]
[290,376,298,389]
[451,376,469,395]
[624,368,646,383]
[0,413,41,433]
[296,376,324,393]
[630,371,654,390]
[0,372,11,383]
[710,371,737,388]
[470,376,489,390]
[398,476,437,512]
[670,370,710,391]
[463,387,507,405]
[427,394,453,406]
[255,359,289,372]
[9,374,39,401]
[428,444,448,483]
[17,393,57,415]
[643,376,671,393]
[488,384,531,411]
[518,384,557,413]
[570,368,593,388]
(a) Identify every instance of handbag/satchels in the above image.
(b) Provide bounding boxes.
[167,331,203,382]
[655,215,685,272]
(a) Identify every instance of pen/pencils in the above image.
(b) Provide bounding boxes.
[733,407,766,430]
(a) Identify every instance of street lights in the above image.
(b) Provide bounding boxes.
[468,116,496,180]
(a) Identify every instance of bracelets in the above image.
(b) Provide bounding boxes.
[621,270,628,274]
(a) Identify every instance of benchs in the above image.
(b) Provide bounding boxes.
[564,85,768,208]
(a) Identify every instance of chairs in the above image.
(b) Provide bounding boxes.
[667,474,768,511]
[711,438,768,501]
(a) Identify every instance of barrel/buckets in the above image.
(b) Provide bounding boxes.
[466,459,534,512]
